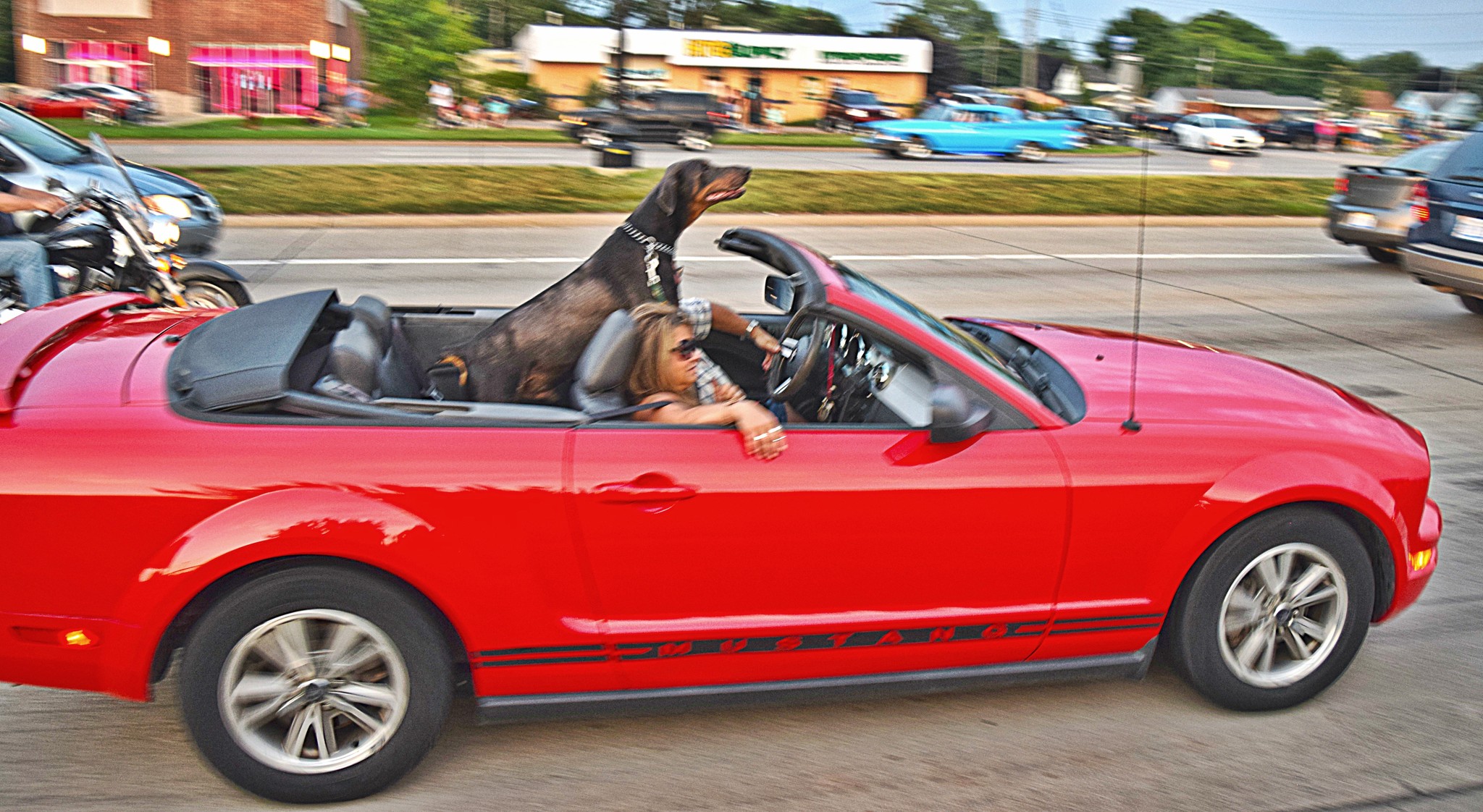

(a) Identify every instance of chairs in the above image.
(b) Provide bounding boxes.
[566,307,641,422]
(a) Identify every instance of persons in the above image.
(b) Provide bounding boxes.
[345,79,374,123]
[0,174,88,312]
[627,259,788,461]
[424,77,511,125]
[1314,114,1336,152]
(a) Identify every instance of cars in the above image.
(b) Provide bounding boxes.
[1400,129,1483,315]
[1323,139,1465,265]
[0,101,226,260]
[0,79,153,121]
[811,82,1483,166]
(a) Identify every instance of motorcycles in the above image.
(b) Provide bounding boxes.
[0,133,255,319]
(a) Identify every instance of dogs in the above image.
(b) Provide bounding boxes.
[453,158,753,410]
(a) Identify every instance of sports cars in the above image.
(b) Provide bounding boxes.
[0,225,1448,806]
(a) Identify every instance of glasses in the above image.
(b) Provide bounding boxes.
[669,338,696,361]
[672,265,684,278]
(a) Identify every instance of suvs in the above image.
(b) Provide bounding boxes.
[559,88,741,151]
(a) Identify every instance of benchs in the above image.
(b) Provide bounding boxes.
[313,295,422,406]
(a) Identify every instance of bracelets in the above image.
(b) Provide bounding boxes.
[745,319,759,340]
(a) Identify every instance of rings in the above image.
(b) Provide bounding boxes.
[753,424,783,440]
